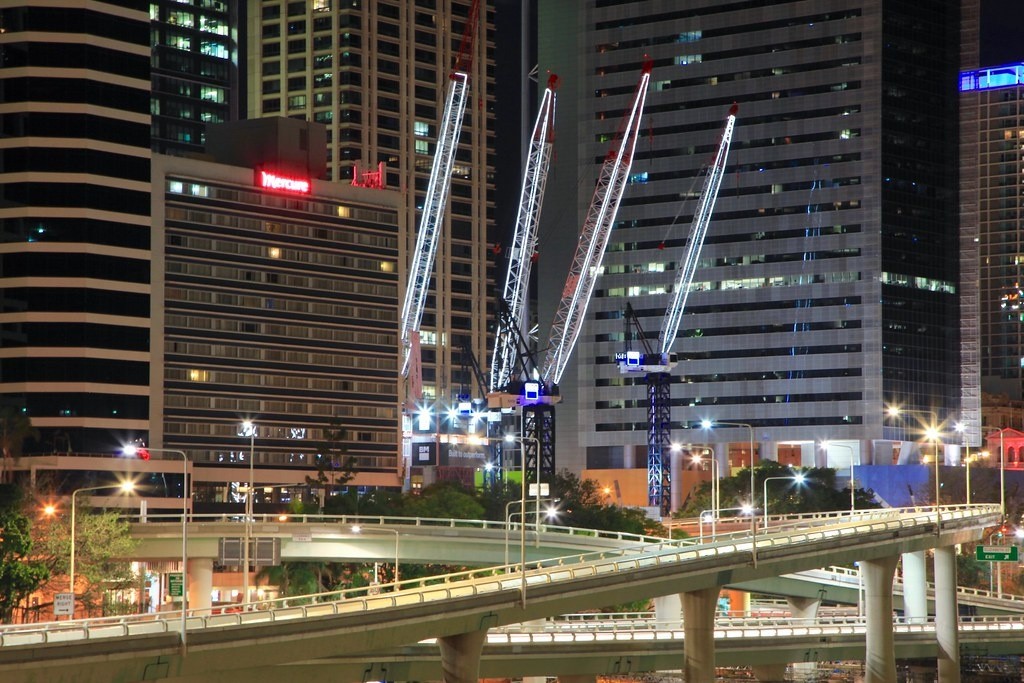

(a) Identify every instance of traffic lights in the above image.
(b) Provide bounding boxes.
[999,526,1006,537]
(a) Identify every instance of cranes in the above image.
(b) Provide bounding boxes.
[394,68,651,496]
[618,101,739,516]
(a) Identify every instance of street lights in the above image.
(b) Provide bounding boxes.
[927,430,970,507]
[820,439,854,516]
[122,444,187,652]
[888,407,940,526]
[954,422,1005,514]
[508,509,557,538]
[68,481,134,616]
[505,498,559,574]
[701,419,757,564]
[242,420,252,534]
[764,476,803,533]
[671,444,716,520]
[990,530,1024,598]
[503,436,540,551]
[690,454,720,519]
[353,527,400,591]
